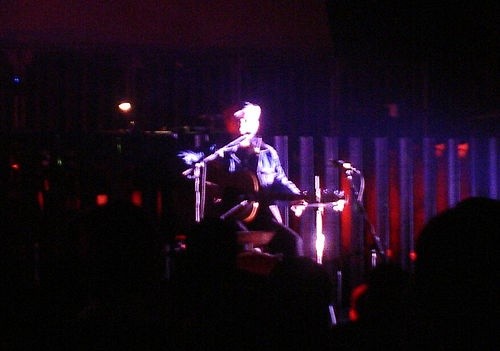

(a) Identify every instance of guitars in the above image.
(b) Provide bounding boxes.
[203,169,348,223]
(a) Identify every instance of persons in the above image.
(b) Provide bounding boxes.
[174,101,308,259]
[1,196,500,351]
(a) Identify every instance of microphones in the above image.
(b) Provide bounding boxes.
[330,159,361,176]
